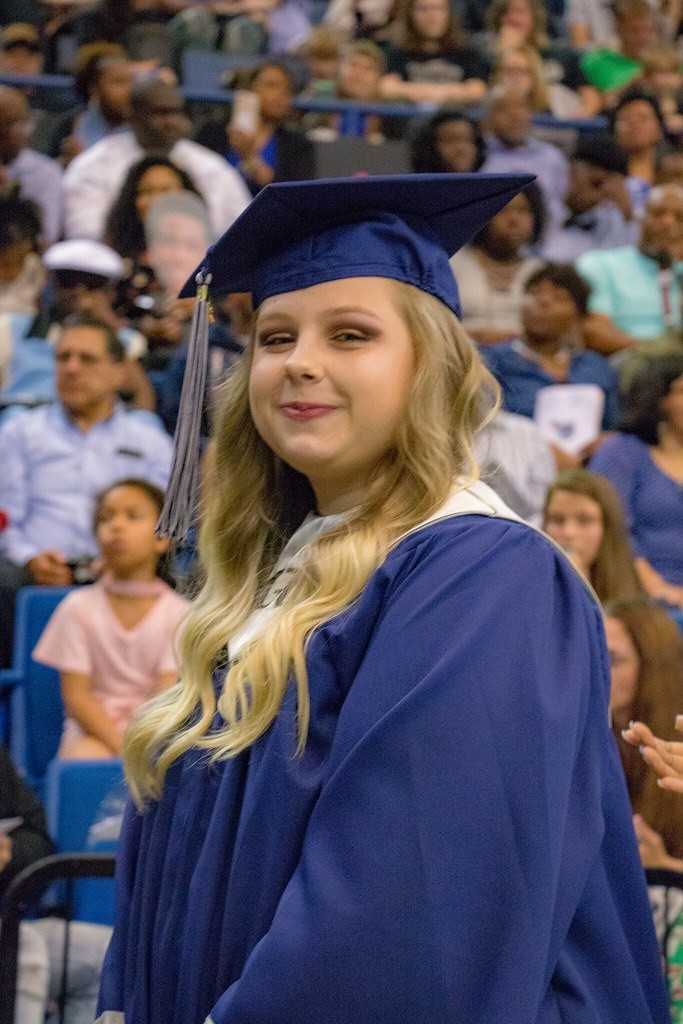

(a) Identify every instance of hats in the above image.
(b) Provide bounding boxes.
[146,173,537,540]
[41,237,125,287]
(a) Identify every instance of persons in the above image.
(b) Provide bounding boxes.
[93,167,666,1024]
[0,0,683,878]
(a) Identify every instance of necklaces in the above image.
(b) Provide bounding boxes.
[103,576,161,596]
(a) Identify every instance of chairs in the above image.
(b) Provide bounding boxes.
[12,587,86,787]
[40,761,129,928]
